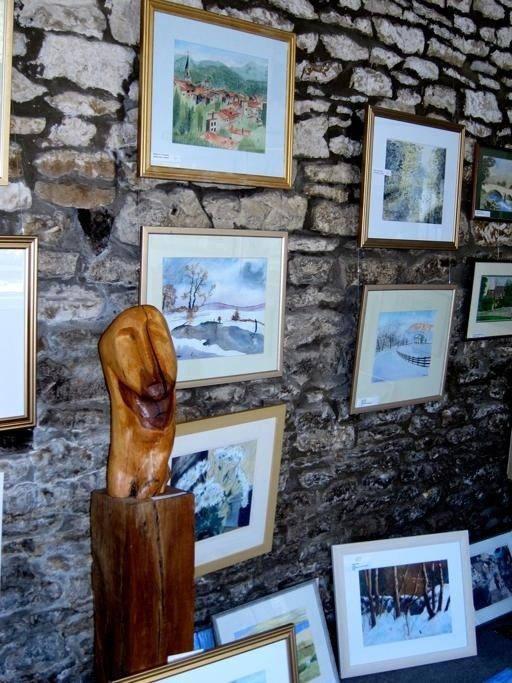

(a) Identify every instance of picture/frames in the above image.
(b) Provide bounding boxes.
[2,236,38,427]
[214,576,340,682]
[470,143,511,222]
[102,621,298,682]
[470,531,512,627]
[359,102,467,251]
[459,259,511,340]
[138,224,289,392]
[166,403,288,580]
[349,283,458,416]
[138,0,298,191]
[331,530,477,679]
